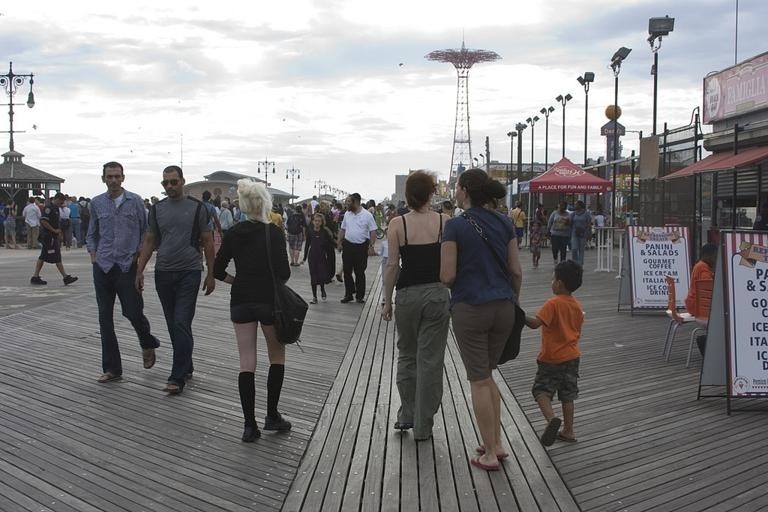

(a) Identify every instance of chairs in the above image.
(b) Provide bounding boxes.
[661,273,696,364]
[685,278,713,368]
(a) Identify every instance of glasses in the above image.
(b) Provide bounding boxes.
[161,179,179,187]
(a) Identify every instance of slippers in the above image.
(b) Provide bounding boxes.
[542,417,560,446]
[471,446,508,471]
[556,431,577,443]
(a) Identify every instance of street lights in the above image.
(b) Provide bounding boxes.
[555,94,573,159]
[474,154,485,166]
[647,15,675,136]
[285,165,300,195]
[576,71,595,208]
[609,46,632,247]
[540,107,554,169]
[314,178,367,203]
[257,156,276,189]
[508,116,541,183]
[0,61,35,151]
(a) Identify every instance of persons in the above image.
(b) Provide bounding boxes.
[524,260,585,446]
[135,165,215,393]
[85,161,161,382]
[0,191,606,304]
[685,243,717,356]
[439,169,522,470]
[212,178,291,442]
[380,169,453,442]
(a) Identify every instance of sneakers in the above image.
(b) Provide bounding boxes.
[290,262,300,266]
[242,423,262,441]
[394,421,414,429]
[143,348,155,369]
[263,411,292,431]
[98,374,122,383]
[309,274,364,304]
[31,276,48,285]
[63,275,78,285]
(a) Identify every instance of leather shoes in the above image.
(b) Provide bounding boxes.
[162,384,183,393]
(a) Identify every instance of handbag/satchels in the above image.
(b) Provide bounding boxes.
[272,283,308,345]
[498,305,525,365]
